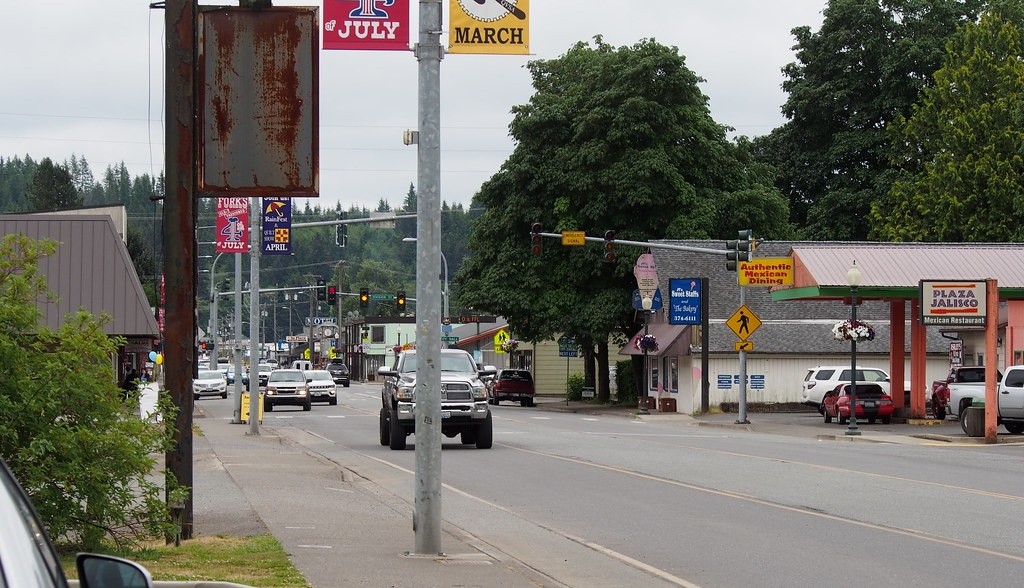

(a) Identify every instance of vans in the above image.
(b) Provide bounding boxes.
[289,360,313,371]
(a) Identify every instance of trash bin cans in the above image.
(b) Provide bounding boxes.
[967,407,985,437]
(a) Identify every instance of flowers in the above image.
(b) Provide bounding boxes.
[634,335,659,352]
[500,339,518,352]
[833,319,875,342]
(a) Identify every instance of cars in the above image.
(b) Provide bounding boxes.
[244,342,270,363]
[192,370,231,400]
[259,357,280,368]
[223,365,248,385]
[478,366,498,388]
[821,382,896,425]
[0,450,153,588]
[198,348,229,370]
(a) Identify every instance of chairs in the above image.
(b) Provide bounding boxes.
[275,374,283,380]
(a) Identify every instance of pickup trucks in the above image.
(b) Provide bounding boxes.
[944,365,1024,438]
[931,366,1005,422]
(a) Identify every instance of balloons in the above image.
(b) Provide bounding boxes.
[156,354,162,364]
[149,351,157,361]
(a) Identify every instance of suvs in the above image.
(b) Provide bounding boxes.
[246,363,274,387]
[377,349,493,449]
[486,368,536,407]
[262,369,312,411]
[802,366,927,416]
[302,369,337,405]
[322,363,350,387]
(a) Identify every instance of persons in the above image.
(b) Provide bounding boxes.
[125,368,149,392]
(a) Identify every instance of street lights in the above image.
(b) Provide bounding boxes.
[637,295,654,416]
[402,237,451,349]
[845,259,864,435]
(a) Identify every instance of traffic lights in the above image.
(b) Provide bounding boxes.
[726,240,738,271]
[327,286,336,306]
[737,230,749,262]
[202,343,214,350]
[531,223,542,256]
[317,283,327,301]
[224,278,231,301]
[396,290,406,312]
[360,288,369,308]
[604,229,616,261]
